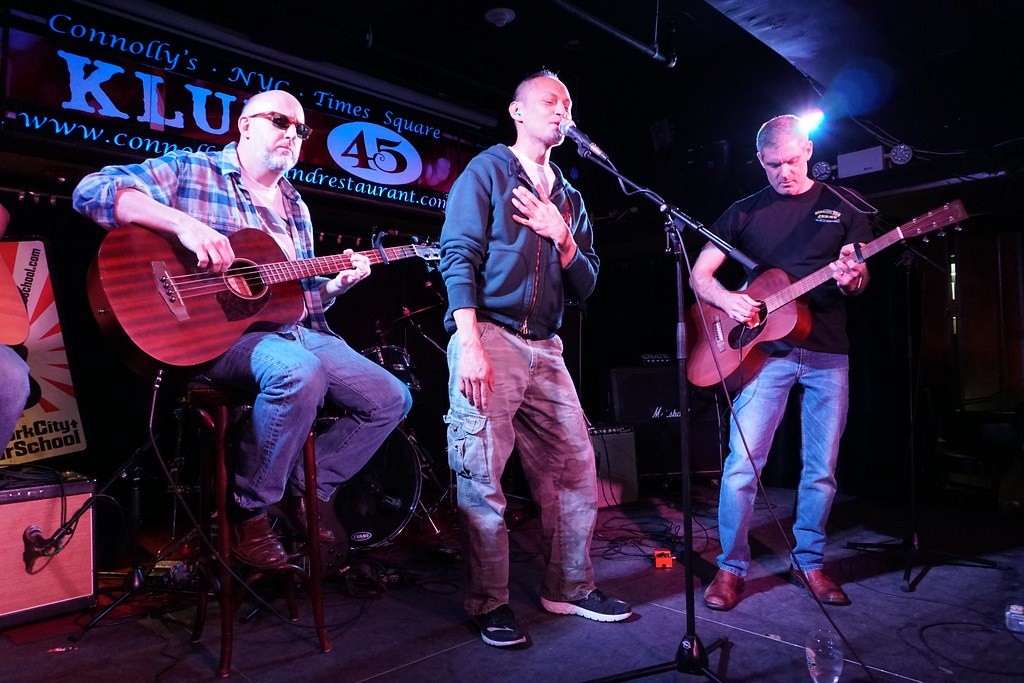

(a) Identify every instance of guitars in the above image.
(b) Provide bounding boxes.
[684,200,971,397]
[85,222,451,376]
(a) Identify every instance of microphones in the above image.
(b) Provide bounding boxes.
[25,524,49,556]
[558,118,609,161]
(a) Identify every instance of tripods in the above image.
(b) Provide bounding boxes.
[576,140,759,683]
[846,220,1013,592]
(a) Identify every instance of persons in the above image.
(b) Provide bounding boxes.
[73,90,412,569]
[438,71,632,645]
[688,113,871,608]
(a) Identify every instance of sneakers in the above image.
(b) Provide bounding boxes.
[475,604,525,646]
[541,589,631,623]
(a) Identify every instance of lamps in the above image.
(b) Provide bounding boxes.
[812,143,915,183]
[485,9,515,25]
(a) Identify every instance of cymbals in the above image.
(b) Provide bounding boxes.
[389,301,450,326]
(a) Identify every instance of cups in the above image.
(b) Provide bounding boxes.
[806,630,843,683]
[1005,601,1024,631]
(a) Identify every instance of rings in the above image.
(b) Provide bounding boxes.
[840,270,843,276]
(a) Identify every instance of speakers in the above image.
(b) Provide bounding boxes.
[589,424,640,509]
[0,467,97,628]
[609,365,723,482]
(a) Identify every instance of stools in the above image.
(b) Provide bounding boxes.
[189,383,338,677]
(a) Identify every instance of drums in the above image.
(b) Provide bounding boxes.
[284,415,422,552]
[357,345,422,401]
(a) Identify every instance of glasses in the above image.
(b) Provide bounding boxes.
[248,111,313,139]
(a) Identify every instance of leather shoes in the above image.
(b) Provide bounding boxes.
[789,563,845,603]
[290,493,346,544]
[230,509,286,568]
[704,568,745,608]
